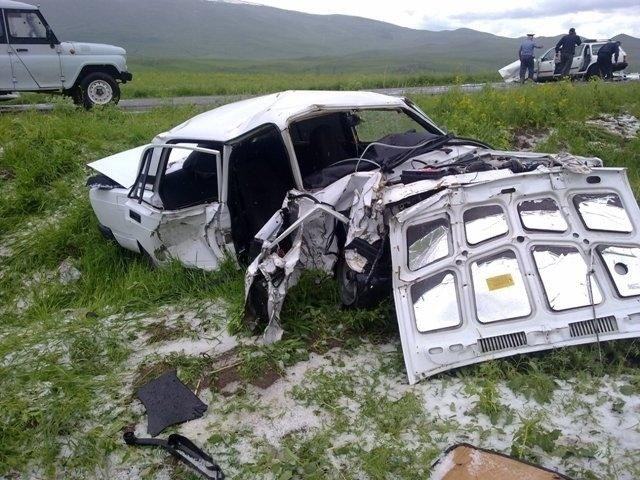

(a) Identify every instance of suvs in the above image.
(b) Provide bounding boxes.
[0,0,133,110]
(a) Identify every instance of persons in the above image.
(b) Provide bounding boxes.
[554,28,584,81]
[597,39,622,83]
[518,32,543,84]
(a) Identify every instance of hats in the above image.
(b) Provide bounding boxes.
[525,33,535,37]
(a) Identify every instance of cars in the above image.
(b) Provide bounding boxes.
[534,39,628,82]
